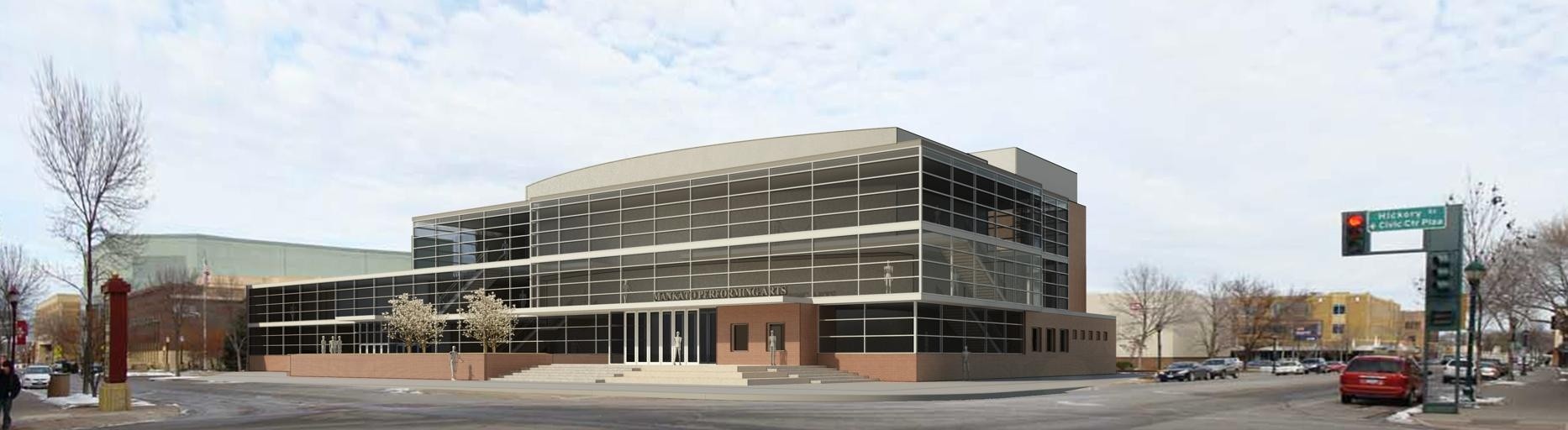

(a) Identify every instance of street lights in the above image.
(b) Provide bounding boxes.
[1511,317,1519,353]
[1521,330,1529,375]
[1461,256,1486,401]
[9,285,19,376]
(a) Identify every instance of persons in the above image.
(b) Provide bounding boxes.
[953,267,962,296]
[518,289,527,308]
[328,336,335,353]
[449,345,463,381]
[289,303,297,321]
[621,279,633,302]
[959,344,971,381]
[0,359,22,429]
[334,335,343,353]
[671,330,682,365]
[883,259,894,293]
[767,329,778,366]
[501,239,509,260]
[320,335,327,354]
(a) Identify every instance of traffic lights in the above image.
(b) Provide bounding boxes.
[1433,254,1451,293]
[1347,214,1365,254]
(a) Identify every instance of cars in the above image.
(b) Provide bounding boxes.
[19,361,51,390]
[1274,358,1347,374]
[1442,356,1510,383]
[1340,354,1426,406]
[1158,357,1244,381]
[54,360,101,373]
